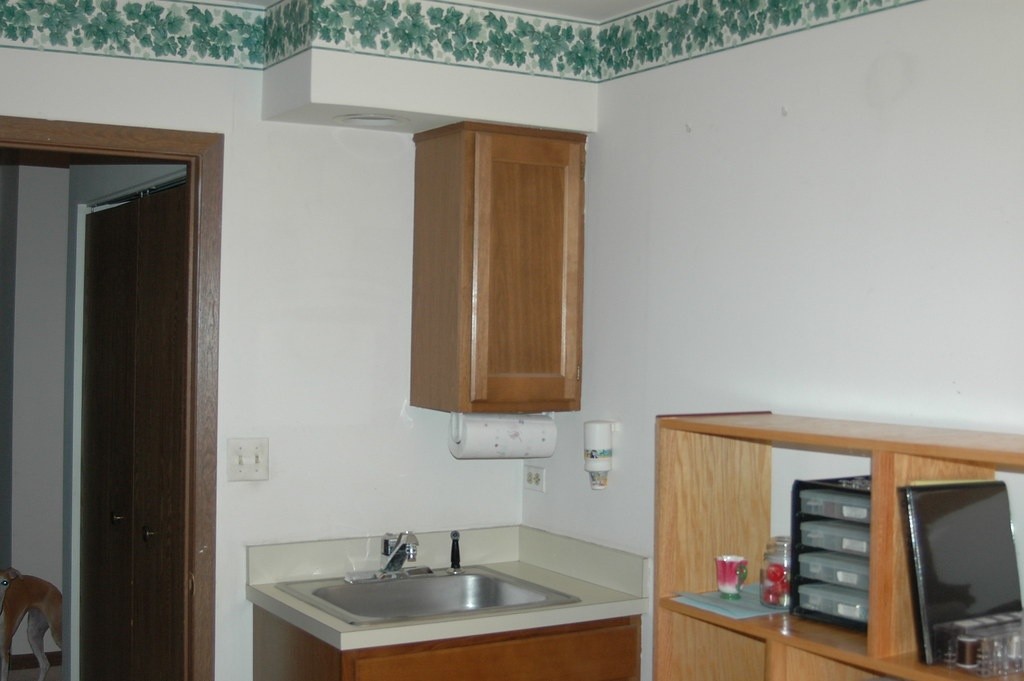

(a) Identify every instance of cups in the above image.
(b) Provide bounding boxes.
[714,555,748,600]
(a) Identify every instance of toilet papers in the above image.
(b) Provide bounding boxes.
[449,414,557,460]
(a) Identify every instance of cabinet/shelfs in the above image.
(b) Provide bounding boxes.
[652,411,1024,680]
[408,121,589,415]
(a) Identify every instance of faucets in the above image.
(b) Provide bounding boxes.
[382,531,417,571]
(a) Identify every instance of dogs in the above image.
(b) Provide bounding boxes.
[0,568,62,681]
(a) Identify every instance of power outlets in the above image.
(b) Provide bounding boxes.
[225,436,272,483]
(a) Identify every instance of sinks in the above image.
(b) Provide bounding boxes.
[275,565,581,625]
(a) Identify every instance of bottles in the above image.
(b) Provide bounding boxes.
[759,537,792,609]
